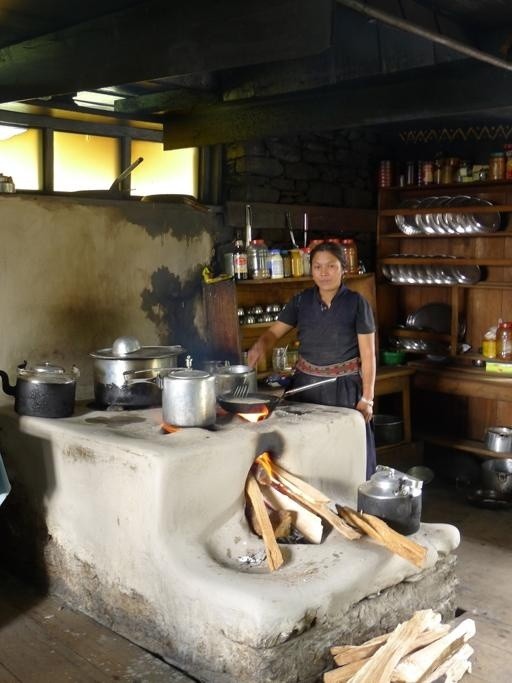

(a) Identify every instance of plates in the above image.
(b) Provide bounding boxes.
[382,252,481,286]
[394,196,502,235]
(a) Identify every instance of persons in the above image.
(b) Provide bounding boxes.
[246,242,379,480]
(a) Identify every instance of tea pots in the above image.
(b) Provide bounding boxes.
[0,359,81,418]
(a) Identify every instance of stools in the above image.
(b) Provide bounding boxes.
[369,364,417,442]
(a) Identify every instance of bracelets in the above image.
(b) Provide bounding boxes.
[361,396,374,407]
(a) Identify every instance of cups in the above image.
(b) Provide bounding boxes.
[272,347,287,371]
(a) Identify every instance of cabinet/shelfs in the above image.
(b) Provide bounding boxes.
[402,364,512,508]
[375,180,512,363]
[200,271,381,380]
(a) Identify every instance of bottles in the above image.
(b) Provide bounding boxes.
[303,247,310,276]
[489,153,506,183]
[506,152,512,182]
[327,239,345,274]
[422,162,433,184]
[234,240,248,281]
[272,348,285,371]
[291,251,303,277]
[307,240,325,249]
[269,250,283,279]
[280,250,292,277]
[482,336,495,358]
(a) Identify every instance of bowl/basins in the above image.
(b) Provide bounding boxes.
[382,351,405,365]
[236,303,289,325]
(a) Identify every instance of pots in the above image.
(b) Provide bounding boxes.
[88,346,187,408]
[482,426,512,453]
[357,464,424,536]
[480,458,512,493]
[213,365,257,397]
[217,377,337,415]
[122,355,216,427]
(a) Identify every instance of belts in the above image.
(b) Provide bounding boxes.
[296,356,362,377]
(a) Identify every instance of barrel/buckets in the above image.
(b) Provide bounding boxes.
[327,238,341,243]
[307,240,325,251]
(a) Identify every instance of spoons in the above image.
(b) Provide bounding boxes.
[111,335,181,355]
